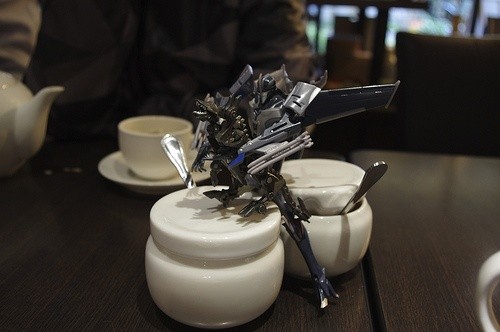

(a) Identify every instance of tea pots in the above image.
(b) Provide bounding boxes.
[0,71,64,176]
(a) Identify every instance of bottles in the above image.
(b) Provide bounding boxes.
[273,158,373,282]
[146,186,285,329]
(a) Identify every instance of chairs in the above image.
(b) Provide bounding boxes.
[395,31,500,155]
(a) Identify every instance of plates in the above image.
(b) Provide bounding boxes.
[98,150,216,196]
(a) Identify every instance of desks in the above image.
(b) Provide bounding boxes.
[350,148,500,332]
[0,141,375,332]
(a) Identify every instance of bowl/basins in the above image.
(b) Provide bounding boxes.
[117,116,193,181]
